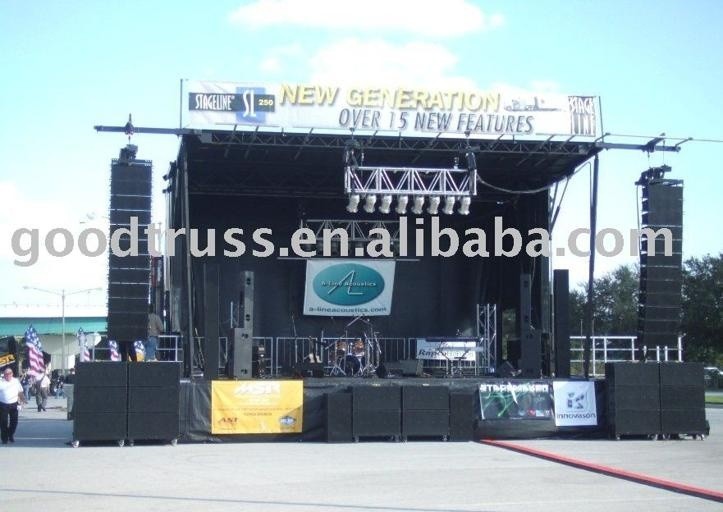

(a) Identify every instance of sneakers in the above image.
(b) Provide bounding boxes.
[38,405,41,412]
[3,435,8,444]
[8,433,14,442]
[41,406,46,411]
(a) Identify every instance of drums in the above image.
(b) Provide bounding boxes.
[337,353,360,375]
[336,341,347,355]
[349,339,364,356]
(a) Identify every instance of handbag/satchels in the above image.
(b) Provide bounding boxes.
[35,381,41,390]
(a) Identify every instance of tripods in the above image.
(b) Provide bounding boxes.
[328,317,383,377]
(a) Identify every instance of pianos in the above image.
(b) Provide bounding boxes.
[426,335,483,342]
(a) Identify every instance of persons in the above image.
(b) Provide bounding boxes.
[33,376,50,412]
[65,368,75,384]
[149,304,164,361]
[0,368,25,444]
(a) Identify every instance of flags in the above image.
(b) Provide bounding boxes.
[24,324,45,377]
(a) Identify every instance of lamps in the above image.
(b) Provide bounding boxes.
[345,193,474,216]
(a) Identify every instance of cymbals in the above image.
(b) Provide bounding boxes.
[370,332,383,337]
[317,341,329,347]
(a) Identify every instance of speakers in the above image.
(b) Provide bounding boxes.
[381,359,424,378]
[73,362,128,447]
[295,363,325,377]
[323,393,352,440]
[401,383,449,441]
[659,361,706,440]
[554,270,570,378]
[450,393,473,441]
[520,274,541,379]
[348,384,402,441]
[229,270,254,379]
[128,362,179,447]
[203,263,218,379]
[605,362,661,440]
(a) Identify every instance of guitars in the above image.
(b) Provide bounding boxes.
[308,336,322,364]
